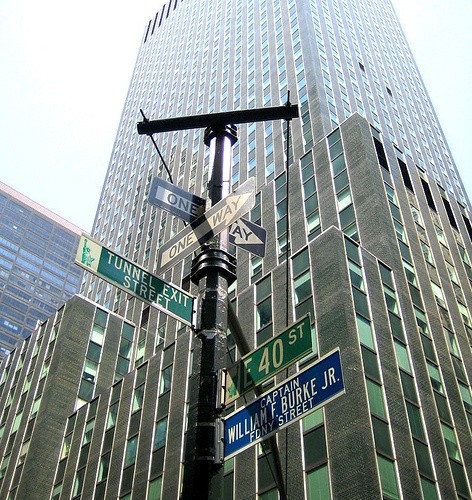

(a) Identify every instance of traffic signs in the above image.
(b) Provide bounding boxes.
[73,233,197,331]
[146,176,267,259]
[156,176,256,277]
[220,312,313,406]
[222,346,347,463]
